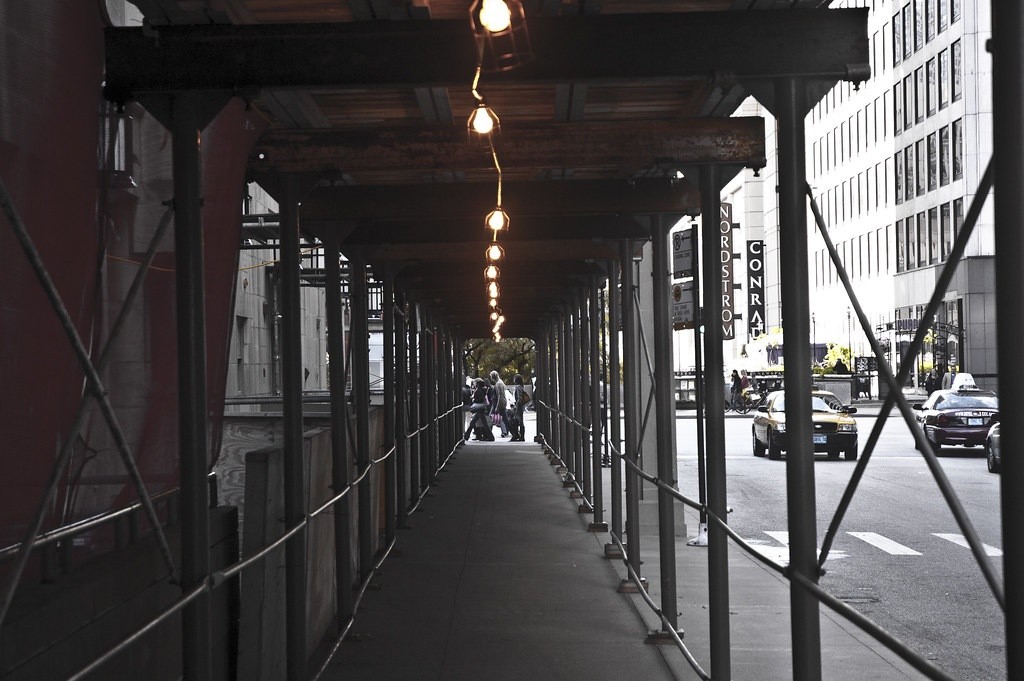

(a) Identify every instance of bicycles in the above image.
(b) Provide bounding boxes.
[718,381,779,414]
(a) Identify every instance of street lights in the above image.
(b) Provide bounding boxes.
[846,307,852,373]
[811,312,817,371]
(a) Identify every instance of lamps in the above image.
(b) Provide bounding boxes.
[469,0,524,36]
[469,105,499,134]
[480,207,506,344]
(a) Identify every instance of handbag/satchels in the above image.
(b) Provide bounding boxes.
[469,403,486,412]
[517,386,531,405]
[490,412,503,425]
[475,415,493,427]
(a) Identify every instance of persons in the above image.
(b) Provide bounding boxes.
[925,369,941,397]
[463,370,525,442]
[724,369,757,412]
[599,373,604,429]
[833,359,848,374]
[942,365,957,389]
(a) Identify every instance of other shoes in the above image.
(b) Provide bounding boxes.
[464,431,520,441]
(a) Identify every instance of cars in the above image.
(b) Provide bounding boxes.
[911,373,1002,456]
[984,412,1003,472]
[751,385,862,461]
